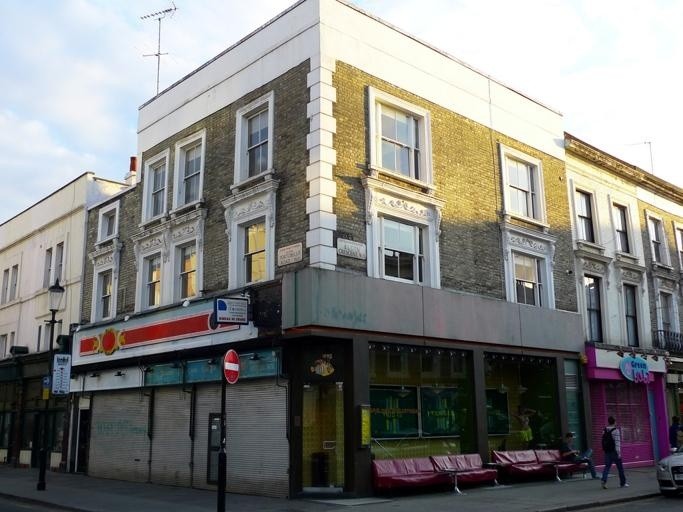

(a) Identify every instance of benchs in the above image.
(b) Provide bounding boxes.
[372,450,589,495]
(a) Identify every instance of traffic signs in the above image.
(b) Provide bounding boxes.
[43,377,50,386]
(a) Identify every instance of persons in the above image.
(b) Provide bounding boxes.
[511,406,536,450]
[670,416,683,448]
[560,432,599,480]
[601,416,629,489]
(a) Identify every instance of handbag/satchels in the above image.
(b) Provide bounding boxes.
[677,431,683,447]
[569,448,579,457]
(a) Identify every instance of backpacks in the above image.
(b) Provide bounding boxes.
[602,428,617,452]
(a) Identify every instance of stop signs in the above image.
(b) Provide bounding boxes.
[224,349,240,385]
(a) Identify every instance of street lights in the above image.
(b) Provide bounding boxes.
[35,279,65,492]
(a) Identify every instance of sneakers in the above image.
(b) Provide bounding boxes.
[601,481,608,489]
[592,475,600,479]
[584,457,589,460]
[624,484,629,487]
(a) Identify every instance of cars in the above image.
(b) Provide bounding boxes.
[657,446,682,498]
